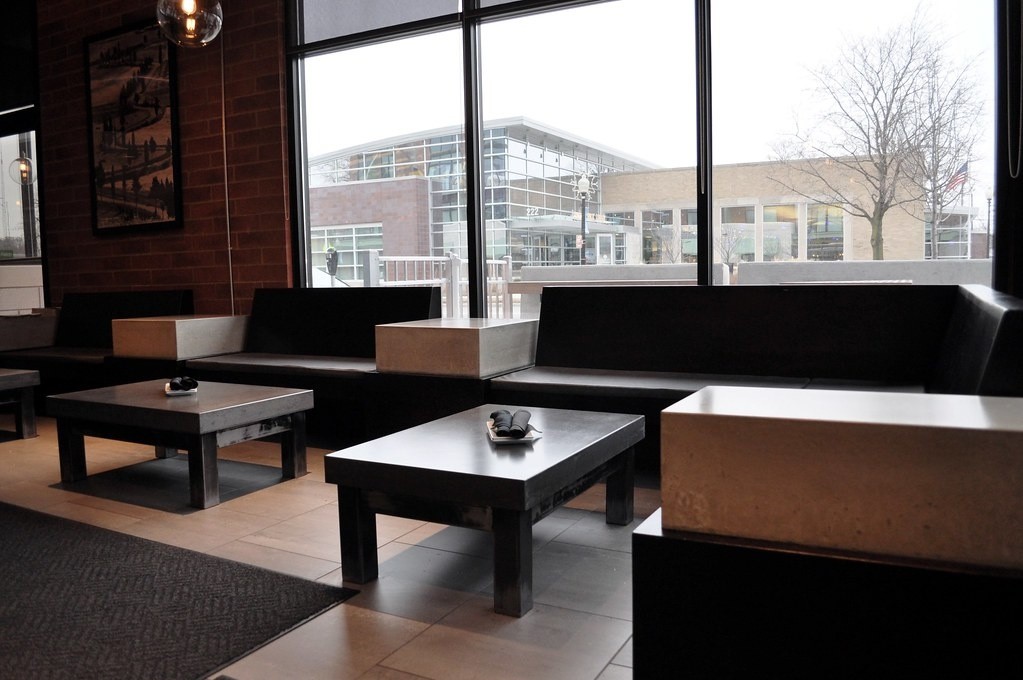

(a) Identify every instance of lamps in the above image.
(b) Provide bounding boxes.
[155,0,224,49]
[8,135,36,186]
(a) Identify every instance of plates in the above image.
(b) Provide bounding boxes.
[165,383,198,396]
[487,421,533,445]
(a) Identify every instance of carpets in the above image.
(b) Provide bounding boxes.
[0,498,361,680]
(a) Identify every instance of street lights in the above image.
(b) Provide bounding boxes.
[570,171,595,265]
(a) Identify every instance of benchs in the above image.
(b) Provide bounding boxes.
[491,285,1022,679]
[5,288,194,420]
[187,287,441,450]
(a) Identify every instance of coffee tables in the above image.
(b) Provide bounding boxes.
[0,367,40,441]
[44,379,315,506]
[321,402,645,617]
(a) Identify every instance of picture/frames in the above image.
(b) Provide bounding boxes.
[82,17,186,237]
